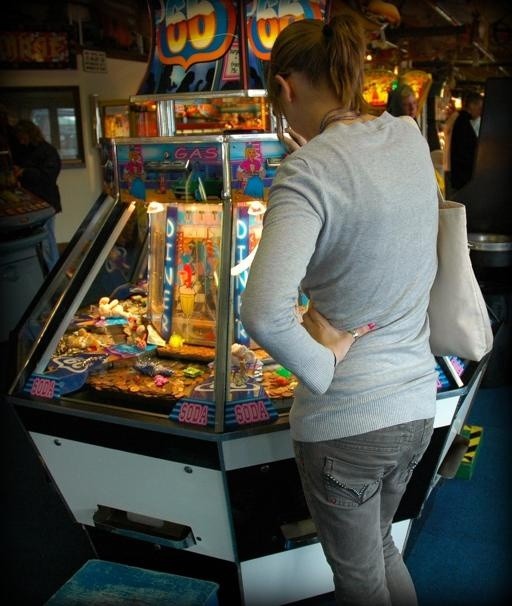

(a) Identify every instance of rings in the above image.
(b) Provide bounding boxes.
[350,330,359,341]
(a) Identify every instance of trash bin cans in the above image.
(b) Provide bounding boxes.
[467,232,512,390]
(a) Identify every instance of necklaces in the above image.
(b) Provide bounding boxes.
[315,113,359,128]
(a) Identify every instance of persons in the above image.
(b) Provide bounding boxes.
[11,119,62,274]
[386,85,418,118]
[239,12,440,604]
[451,91,485,189]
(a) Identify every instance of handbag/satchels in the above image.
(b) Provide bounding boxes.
[426,200,494,362]
